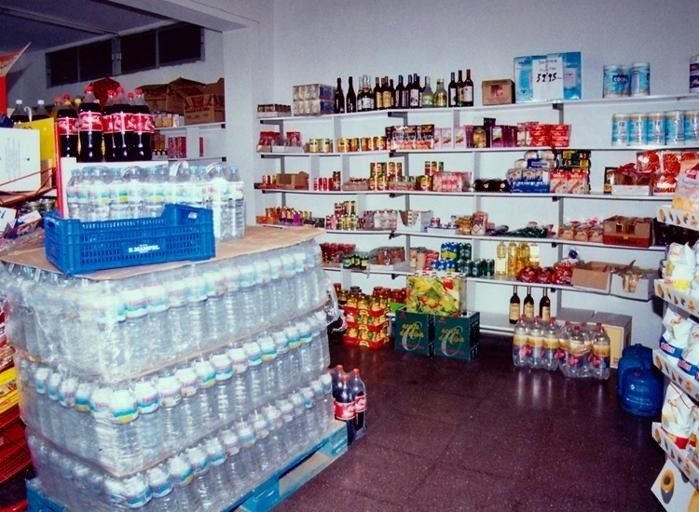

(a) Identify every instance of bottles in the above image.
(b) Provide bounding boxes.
[389,80,395,107]
[383,76,392,109]
[390,79,393,107]
[495,241,506,277]
[342,297,390,350]
[310,138,318,152]
[346,76,357,112]
[508,285,521,324]
[324,178,327,189]
[105,89,119,162]
[168,136,177,157]
[154,133,165,152]
[333,282,366,304]
[333,171,340,190]
[328,178,334,191]
[540,286,551,321]
[425,162,430,175]
[10,100,31,129]
[369,162,401,189]
[79,84,104,164]
[129,87,152,160]
[319,138,329,153]
[394,75,407,106]
[439,162,443,171]
[524,287,534,322]
[354,256,360,267]
[337,138,349,152]
[508,241,518,277]
[9,309,337,512]
[112,87,138,162]
[631,62,651,96]
[33,100,52,120]
[448,73,457,108]
[343,256,352,269]
[373,136,386,149]
[313,178,317,191]
[668,112,680,146]
[462,68,474,105]
[464,263,471,275]
[441,243,472,261]
[374,76,384,110]
[624,69,631,95]
[333,77,346,114]
[631,114,645,147]
[432,80,447,108]
[685,112,699,143]
[432,161,436,172]
[362,74,371,111]
[56,92,78,157]
[154,113,181,128]
[374,286,407,303]
[456,261,463,272]
[67,168,245,244]
[646,113,666,146]
[349,369,367,433]
[319,178,322,189]
[562,321,611,381]
[331,364,349,382]
[521,243,531,269]
[361,258,369,270]
[381,77,382,82]
[611,114,631,146]
[511,314,564,372]
[361,138,371,152]
[422,77,434,107]
[177,136,187,158]
[458,70,462,106]
[603,65,624,97]
[471,261,481,277]
[350,138,358,152]
[408,75,413,87]
[351,256,355,265]
[430,259,456,272]
[433,171,469,193]
[0,238,329,385]
[409,74,421,107]
[485,259,493,277]
[334,375,355,448]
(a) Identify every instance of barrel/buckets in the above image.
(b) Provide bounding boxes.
[616,352,646,397]
[622,344,652,371]
[621,364,660,417]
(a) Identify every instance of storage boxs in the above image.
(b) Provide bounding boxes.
[142,82,182,114]
[603,216,653,248]
[405,268,467,316]
[182,93,224,125]
[432,309,480,360]
[592,308,632,367]
[554,305,593,326]
[610,269,661,301]
[391,300,432,356]
[571,261,639,294]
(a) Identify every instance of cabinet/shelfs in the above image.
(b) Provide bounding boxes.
[257,94,699,363]
[0,103,227,512]
[648,139,699,512]
